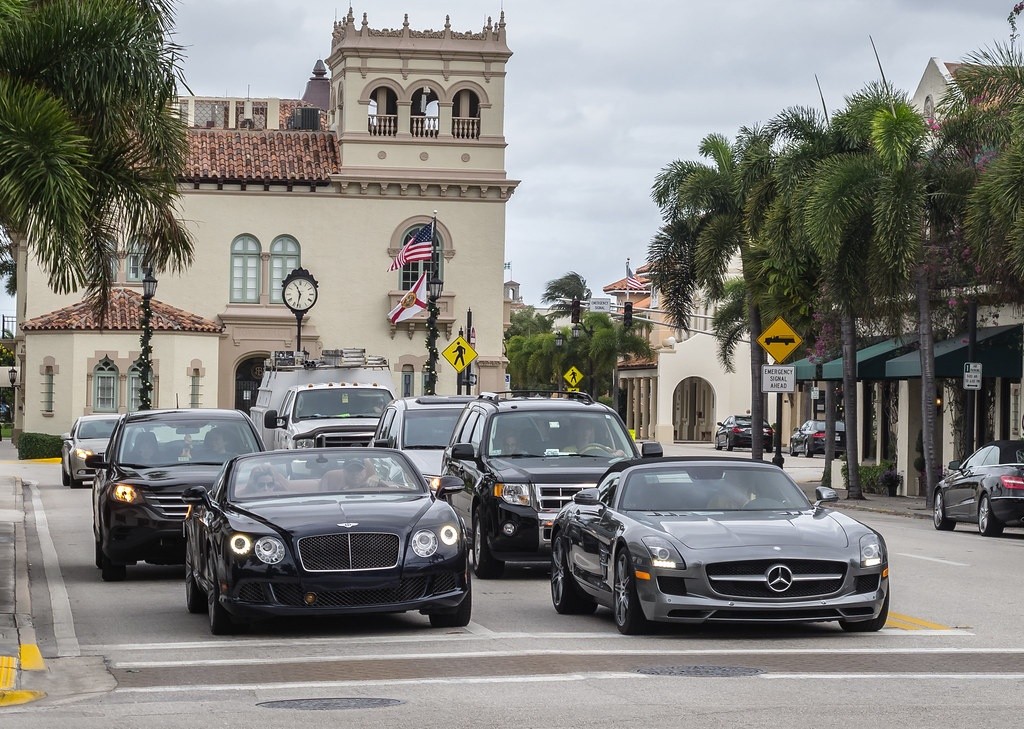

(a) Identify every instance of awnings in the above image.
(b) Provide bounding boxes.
[788,331,947,381]
[886,325,1022,380]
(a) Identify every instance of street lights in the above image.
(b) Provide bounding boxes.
[140,269,159,411]
[426,270,444,395]
[554,322,593,397]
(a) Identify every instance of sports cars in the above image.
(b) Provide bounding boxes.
[549,456,889,636]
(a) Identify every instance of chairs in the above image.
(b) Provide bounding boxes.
[128,432,163,462]
[78,423,97,439]
[520,430,547,454]
[321,470,348,492]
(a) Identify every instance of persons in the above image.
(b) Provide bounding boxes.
[706,471,787,509]
[497,431,528,454]
[244,464,275,494]
[208,427,237,456]
[340,457,382,490]
[562,422,625,457]
[134,437,156,464]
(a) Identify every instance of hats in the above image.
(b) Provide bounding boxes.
[343,457,366,469]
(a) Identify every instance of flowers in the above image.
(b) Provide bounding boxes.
[880,471,904,484]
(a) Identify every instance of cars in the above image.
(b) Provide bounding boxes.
[932,440,1024,537]
[181,447,471,631]
[789,419,845,458]
[60,415,146,488]
[716,415,773,453]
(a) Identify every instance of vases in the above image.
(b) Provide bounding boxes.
[889,483,896,497]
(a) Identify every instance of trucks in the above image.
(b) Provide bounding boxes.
[249,349,400,476]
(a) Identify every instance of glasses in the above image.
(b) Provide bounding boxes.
[257,482,274,489]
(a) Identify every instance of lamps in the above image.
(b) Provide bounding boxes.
[8,365,21,389]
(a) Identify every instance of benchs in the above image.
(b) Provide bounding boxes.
[642,481,720,509]
[237,476,321,493]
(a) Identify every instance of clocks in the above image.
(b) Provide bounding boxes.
[285,277,317,311]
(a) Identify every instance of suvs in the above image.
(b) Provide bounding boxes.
[440,393,665,580]
[366,396,497,495]
[86,410,288,582]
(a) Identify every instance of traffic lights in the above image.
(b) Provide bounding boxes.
[624,302,633,328]
[571,298,580,323]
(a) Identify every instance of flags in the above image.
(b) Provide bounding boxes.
[386,219,434,272]
[626,262,645,290]
[387,270,427,325]
